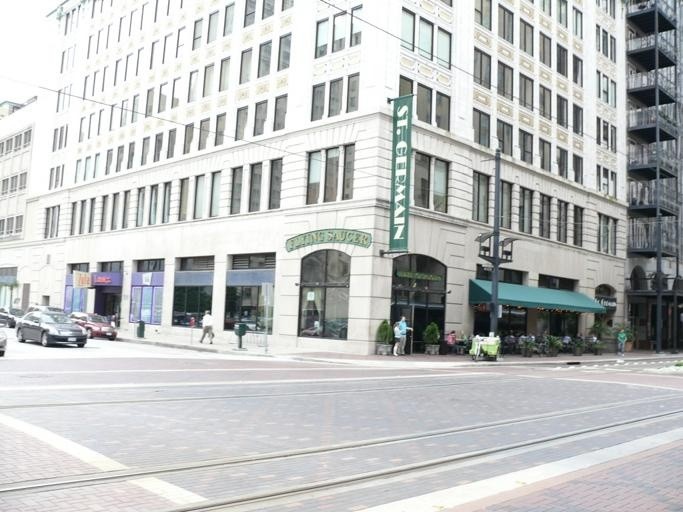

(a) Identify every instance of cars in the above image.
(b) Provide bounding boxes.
[0,304,117,356]
[171,306,318,338]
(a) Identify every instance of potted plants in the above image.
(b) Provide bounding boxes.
[518,332,608,358]
[611,321,637,352]
[375,316,443,359]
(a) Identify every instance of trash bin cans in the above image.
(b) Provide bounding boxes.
[136,320,145,337]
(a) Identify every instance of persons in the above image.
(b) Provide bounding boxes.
[393,322,401,356]
[199,310,214,344]
[446,329,597,354]
[617,329,627,357]
[398,316,413,356]
